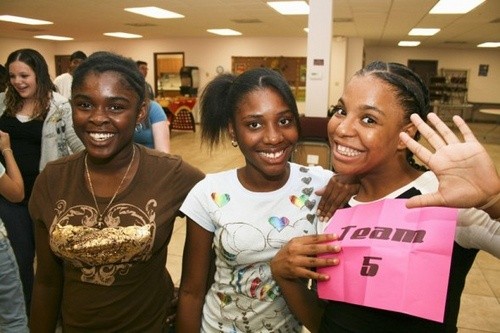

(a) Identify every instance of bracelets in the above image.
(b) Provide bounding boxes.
[477,193,500,210]
[1,148,13,155]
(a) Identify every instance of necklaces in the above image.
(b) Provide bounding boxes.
[85,145,136,228]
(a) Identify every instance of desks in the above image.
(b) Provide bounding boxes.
[479,109,500,140]
[431,104,472,127]
[154,98,196,132]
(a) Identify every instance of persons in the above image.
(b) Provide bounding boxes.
[0,49,216,333]
[175,66,361,333]
[271,62,500,333]
